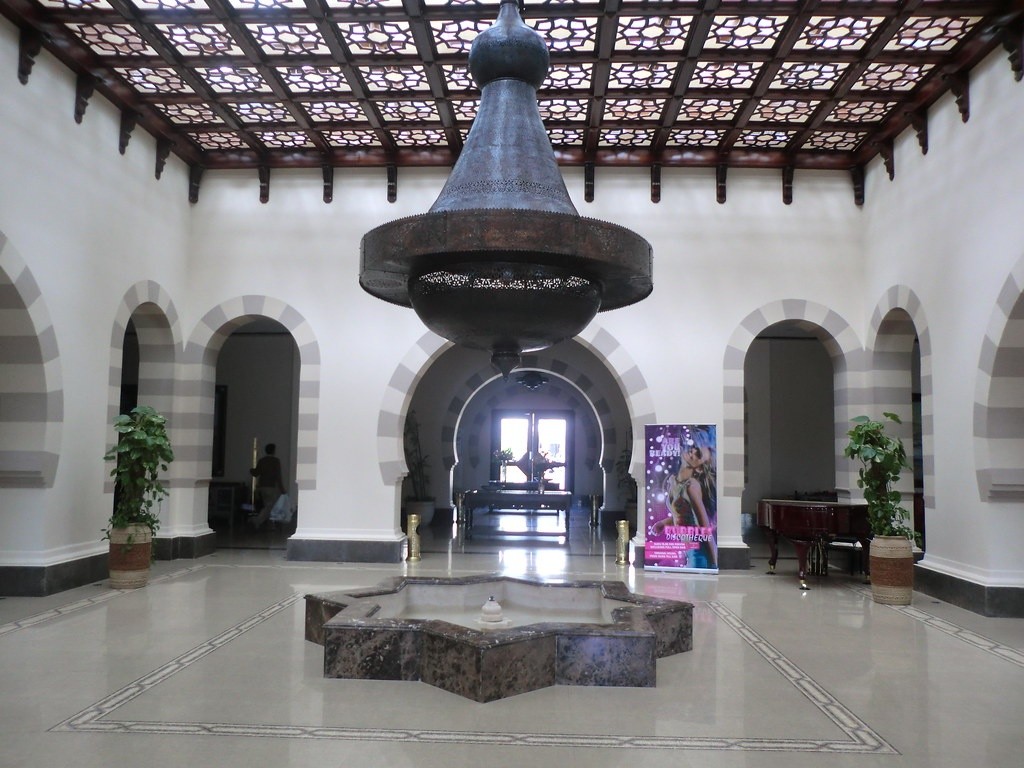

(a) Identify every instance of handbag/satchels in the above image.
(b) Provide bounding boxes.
[270,494,292,523]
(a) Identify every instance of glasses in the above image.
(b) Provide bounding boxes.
[694,447,702,463]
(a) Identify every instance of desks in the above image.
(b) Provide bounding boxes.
[209,481,240,528]
[464,488,572,541]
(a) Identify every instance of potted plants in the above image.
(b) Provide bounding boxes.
[403,410,439,528]
[843,412,923,605]
[617,432,638,530]
[99,405,174,590]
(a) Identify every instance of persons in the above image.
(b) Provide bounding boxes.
[651,444,717,570]
[249,444,290,512]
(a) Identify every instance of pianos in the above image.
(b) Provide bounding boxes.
[756,498,891,592]
